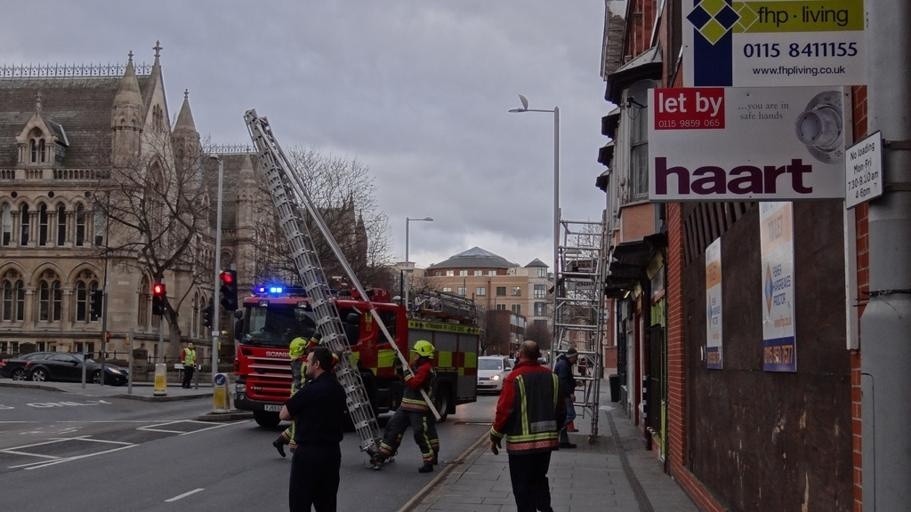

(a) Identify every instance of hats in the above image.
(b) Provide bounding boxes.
[565,347,580,357]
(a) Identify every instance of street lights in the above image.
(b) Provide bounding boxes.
[206,150,222,388]
[508,93,566,343]
[405,216,435,314]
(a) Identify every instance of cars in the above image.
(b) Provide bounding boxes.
[0,348,128,384]
[476,347,551,397]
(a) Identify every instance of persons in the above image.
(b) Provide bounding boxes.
[385,350,440,466]
[270,332,322,458]
[512,347,587,449]
[276,347,347,512]
[490,337,559,511]
[180,341,197,388]
[378,339,437,474]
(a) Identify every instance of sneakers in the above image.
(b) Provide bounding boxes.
[432,446,440,467]
[370,450,386,465]
[418,461,434,474]
[272,436,286,459]
[553,427,579,452]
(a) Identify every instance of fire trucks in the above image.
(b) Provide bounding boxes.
[233,278,481,431]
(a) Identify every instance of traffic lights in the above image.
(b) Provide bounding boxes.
[152,283,166,315]
[200,303,211,329]
[219,268,236,310]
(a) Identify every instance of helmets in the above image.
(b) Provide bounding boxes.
[287,336,307,358]
[413,340,436,360]
